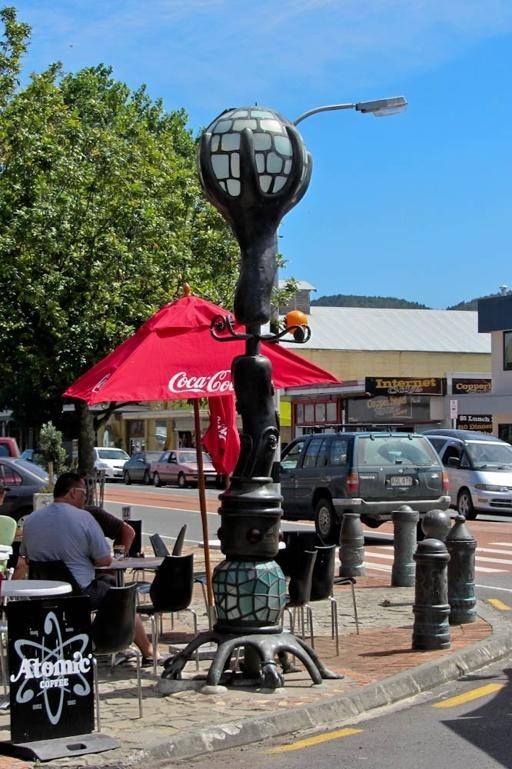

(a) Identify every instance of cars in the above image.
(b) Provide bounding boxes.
[122,450,165,485]
[149,449,221,489]
[1,456,61,522]
[91,446,131,483]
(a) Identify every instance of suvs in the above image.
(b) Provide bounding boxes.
[280,420,452,547]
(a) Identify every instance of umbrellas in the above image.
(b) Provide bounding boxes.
[62,282,344,607]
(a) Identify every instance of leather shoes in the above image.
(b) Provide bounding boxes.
[142,657,165,667]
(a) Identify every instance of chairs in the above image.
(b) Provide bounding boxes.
[92,583,143,731]
[151,533,219,630]
[301,546,340,659]
[133,524,189,635]
[137,556,197,679]
[30,560,83,597]
[272,549,317,655]
[283,530,358,638]
[1,515,23,583]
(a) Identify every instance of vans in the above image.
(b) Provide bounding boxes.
[419,426,512,523]
[1,437,24,487]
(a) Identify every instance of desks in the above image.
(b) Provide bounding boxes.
[2,581,72,709]
[92,558,167,605]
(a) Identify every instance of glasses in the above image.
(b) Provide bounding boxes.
[68,486,89,494]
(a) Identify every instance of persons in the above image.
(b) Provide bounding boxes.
[0,472,175,667]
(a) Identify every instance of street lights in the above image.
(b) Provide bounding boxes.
[264,95,409,516]
